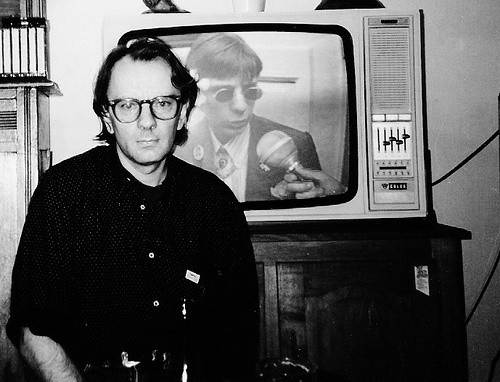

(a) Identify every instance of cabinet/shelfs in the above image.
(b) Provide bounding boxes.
[251,226,472,382]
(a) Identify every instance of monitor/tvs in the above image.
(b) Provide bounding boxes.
[100,7,427,224]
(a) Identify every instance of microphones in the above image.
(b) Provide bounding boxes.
[256,130,312,182]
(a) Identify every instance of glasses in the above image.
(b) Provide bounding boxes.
[199,83,263,102]
[107,95,186,123]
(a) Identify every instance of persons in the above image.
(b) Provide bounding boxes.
[172,32,348,202]
[6,36,260,382]
